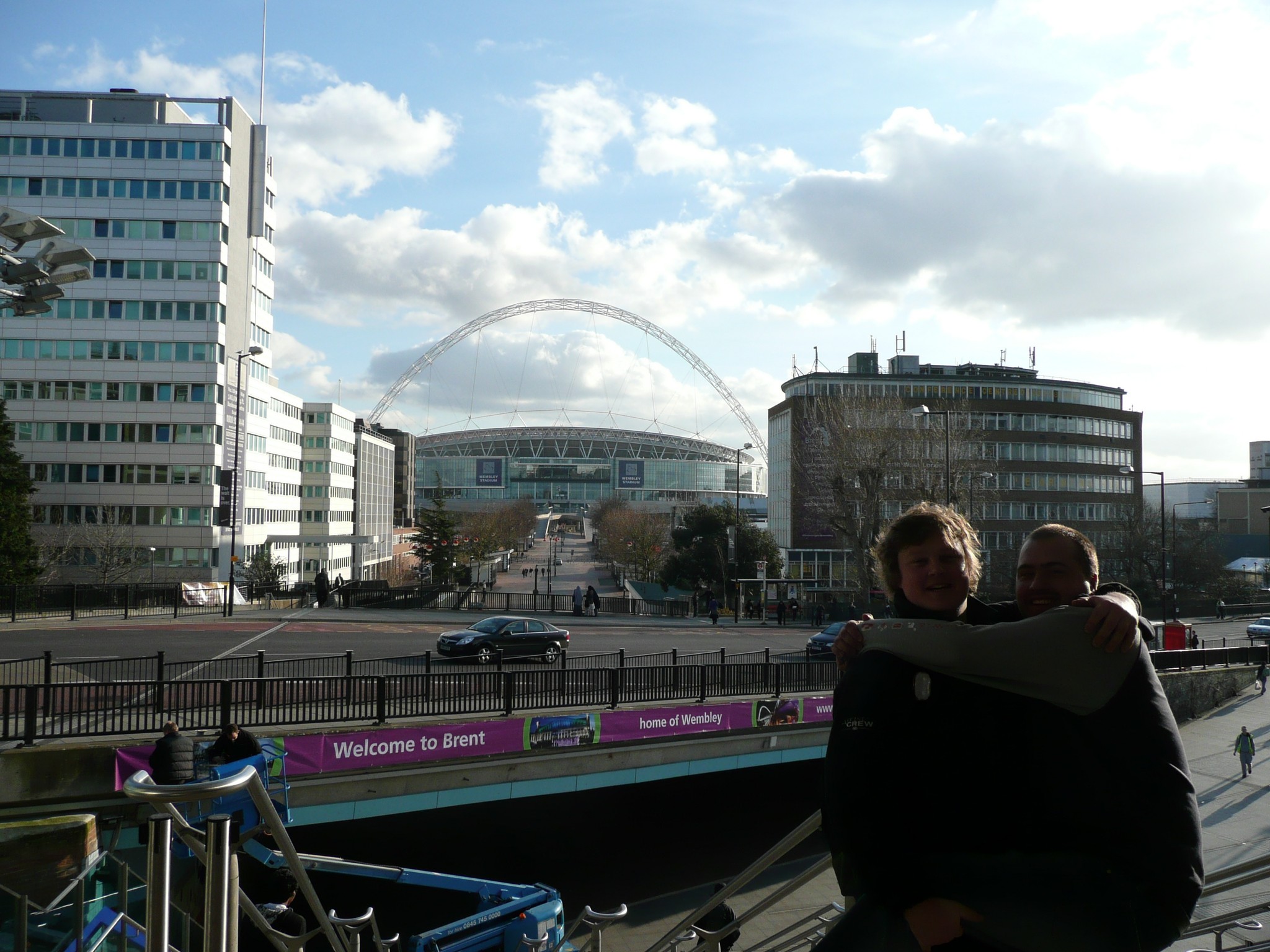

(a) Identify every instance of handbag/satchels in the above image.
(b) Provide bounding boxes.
[1255,680,1261,690]
[584,600,590,608]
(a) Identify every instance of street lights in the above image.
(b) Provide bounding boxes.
[1263,565,1269,589]
[441,537,460,584]
[1119,465,1166,623]
[408,541,433,609]
[228,345,264,616]
[463,535,479,585]
[1253,562,1258,583]
[372,549,380,580]
[910,404,950,509]
[734,442,753,624]
[1173,498,1214,621]
[970,472,993,525]
[627,541,637,581]
[1242,564,1246,580]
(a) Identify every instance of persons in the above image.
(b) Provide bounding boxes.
[1216,597,1225,619]
[822,502,1204,952]
[334,573,343,588]
[481,579,487,602]
[521,565,546,578]
[238,868,308,951]
[1232,726,1256,779]
[1256,662,1268,694]
[690,585,894,629]
[573,584,601,616]
[692,881,740,951]
[314,568,329,609]
[150,720,194,784]
[1191,630,1198,650]
[212,722,262,760]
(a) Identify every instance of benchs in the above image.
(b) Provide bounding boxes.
[767,605,802,613]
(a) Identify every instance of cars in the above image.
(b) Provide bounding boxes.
[1246,617,1270,637]
[436,615,570,665]
[760,601,802,618]
[553,558,562,566]
[806,621,851,657]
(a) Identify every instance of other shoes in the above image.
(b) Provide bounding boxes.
[1264,688,1266,693]
[1260,692,1263,695]
[1248,770,1251,774]
[1242,773,1247,778]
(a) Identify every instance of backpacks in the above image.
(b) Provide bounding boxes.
[1262,667,1270,677]
